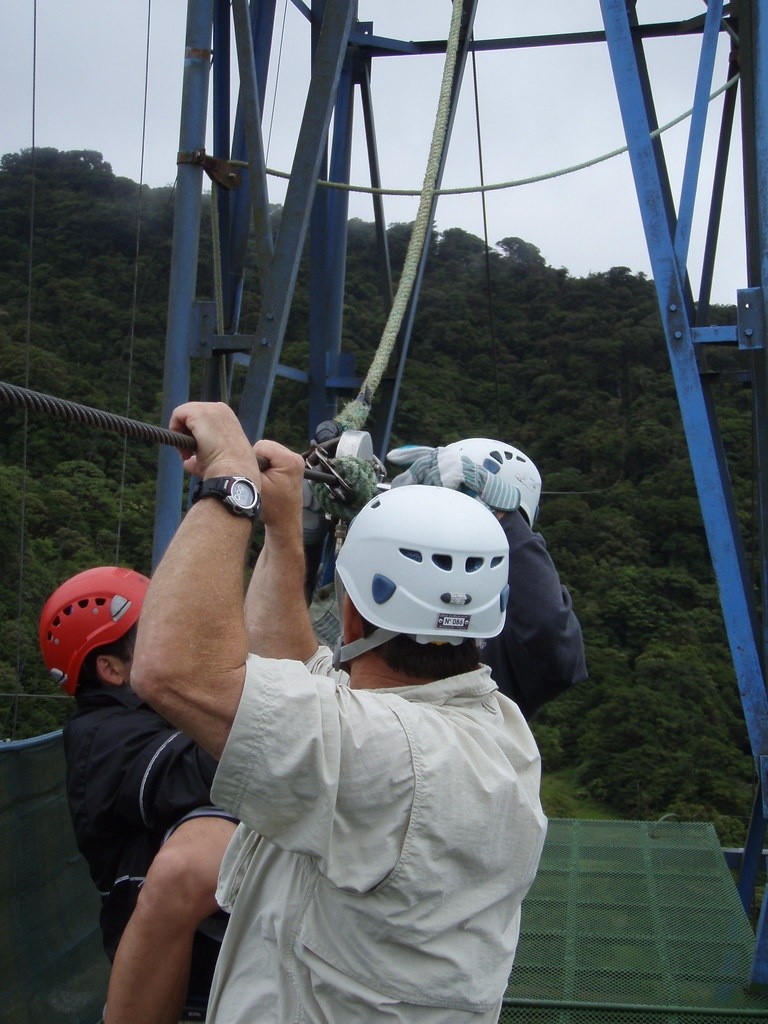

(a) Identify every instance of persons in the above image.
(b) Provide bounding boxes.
[37,437,590,1024]
[103,401,547,1024]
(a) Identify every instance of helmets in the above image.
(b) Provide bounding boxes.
[447,437,542,530]
[39,565,152,697]
[336,483,509,648]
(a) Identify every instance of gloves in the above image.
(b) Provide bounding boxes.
[387,445,521,516]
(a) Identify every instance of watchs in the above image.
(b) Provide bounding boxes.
[191,475,262,526]
